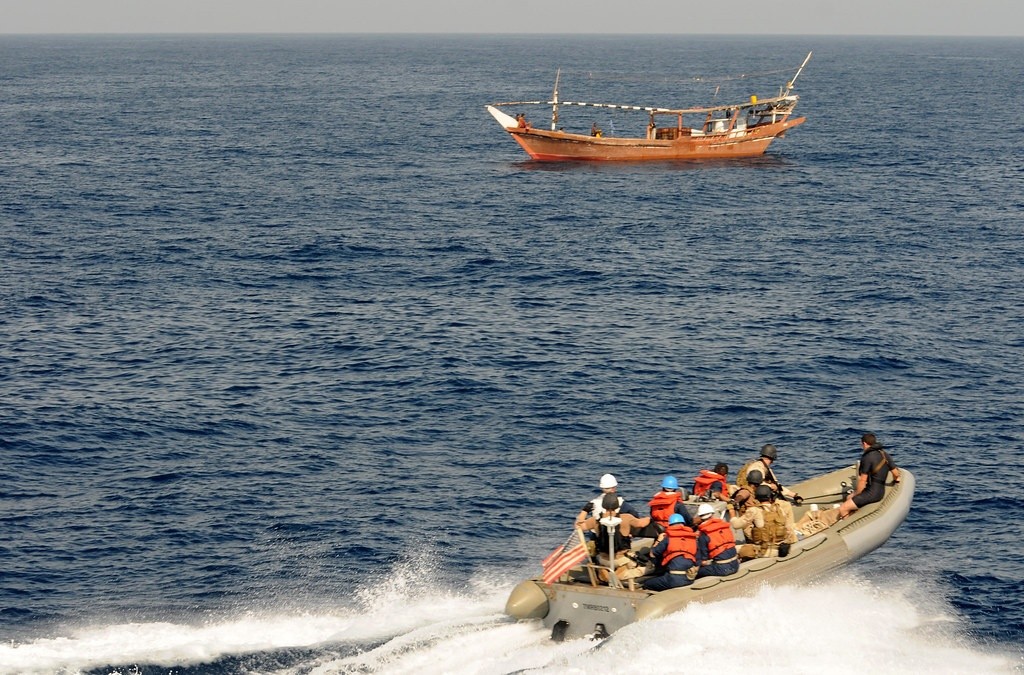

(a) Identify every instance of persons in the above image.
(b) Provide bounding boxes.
[556,127,564,133]
[840,434,899,518]
[693,463,730,501]
[717,444,804,558]
[592,123,597,136]
[574,473,650,581]
[647,476,697,534]
[644,514,694,592]
[649,115,654,127]
[515,113,525,128]
[725,107,732,118]
[694,504,739,579]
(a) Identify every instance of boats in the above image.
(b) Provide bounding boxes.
[481,48,813,165]
[498,459,917,657]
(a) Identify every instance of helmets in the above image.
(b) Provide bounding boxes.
[669,513,685,525]
[697,503,715,516]
[747,470,764,484]
[755,485,772,500]
[601,492,619,510]
[600,474,618,489]
[760,444,776,460]
[660,476,679,489]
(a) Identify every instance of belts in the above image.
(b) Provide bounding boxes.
[717,556,737,564]
[670,570,687,575]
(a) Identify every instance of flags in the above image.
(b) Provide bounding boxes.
[541,529,588,586]
